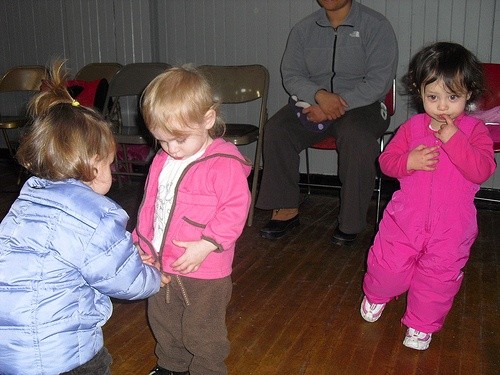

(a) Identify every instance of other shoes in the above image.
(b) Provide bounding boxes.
[148,364,188,375]
[361,296,386,323]
[261,213,300,238]
[402,327,432,350]
[331,224,360,249]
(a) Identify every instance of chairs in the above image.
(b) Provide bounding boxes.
[296,76,396,229]
[0,64,270,228]
[469,64,500,203]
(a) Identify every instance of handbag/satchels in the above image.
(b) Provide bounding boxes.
[39,78,115,116]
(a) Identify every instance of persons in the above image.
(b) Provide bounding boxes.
[360,42,497,350]
[132,64,253,375]
[254,0,398,242]
[0,59,171,375]
